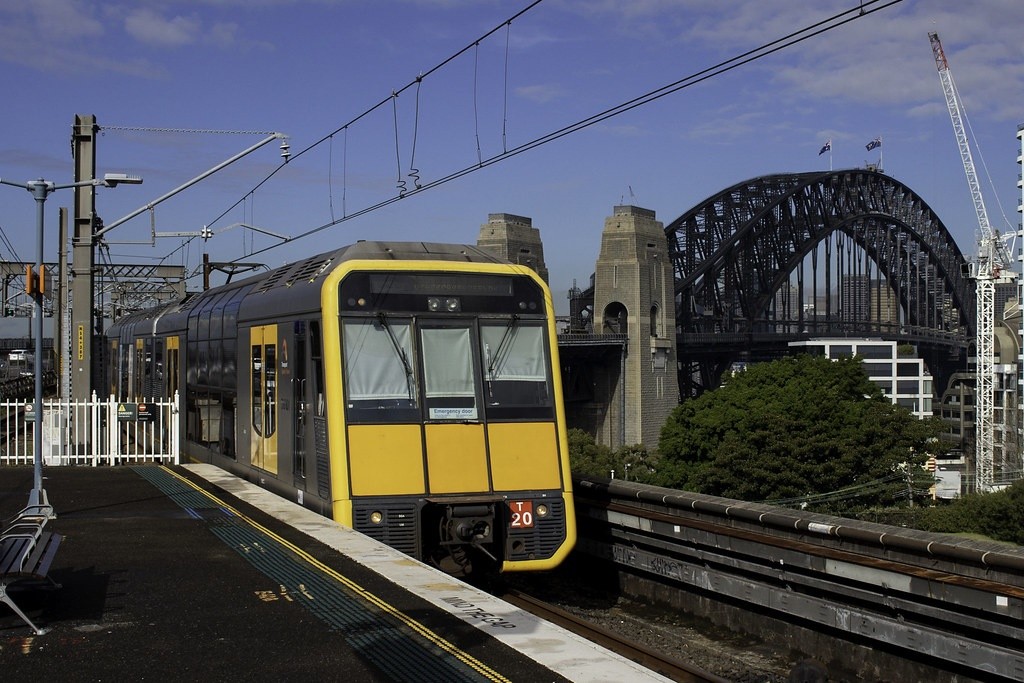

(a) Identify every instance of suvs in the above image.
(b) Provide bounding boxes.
[7,350,34,365]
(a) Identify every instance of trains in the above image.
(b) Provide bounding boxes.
[104,240,577,580]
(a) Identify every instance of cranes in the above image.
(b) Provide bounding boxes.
[927,30,1017,496]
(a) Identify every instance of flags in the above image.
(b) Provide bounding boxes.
[819,140,830,156]
[865,137,881,152]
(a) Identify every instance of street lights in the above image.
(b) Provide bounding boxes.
[0,173,144,515]
